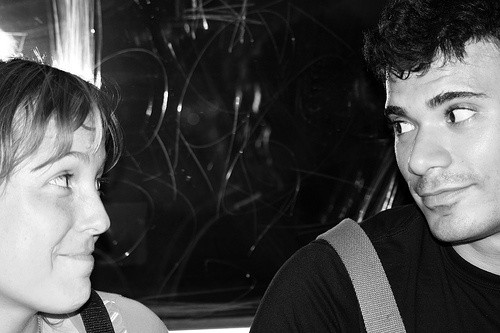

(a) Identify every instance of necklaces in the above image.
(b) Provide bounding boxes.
[36,311,44,332]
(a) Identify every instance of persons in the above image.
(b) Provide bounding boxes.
[0,56,168,332]
[247,1,499,332]
